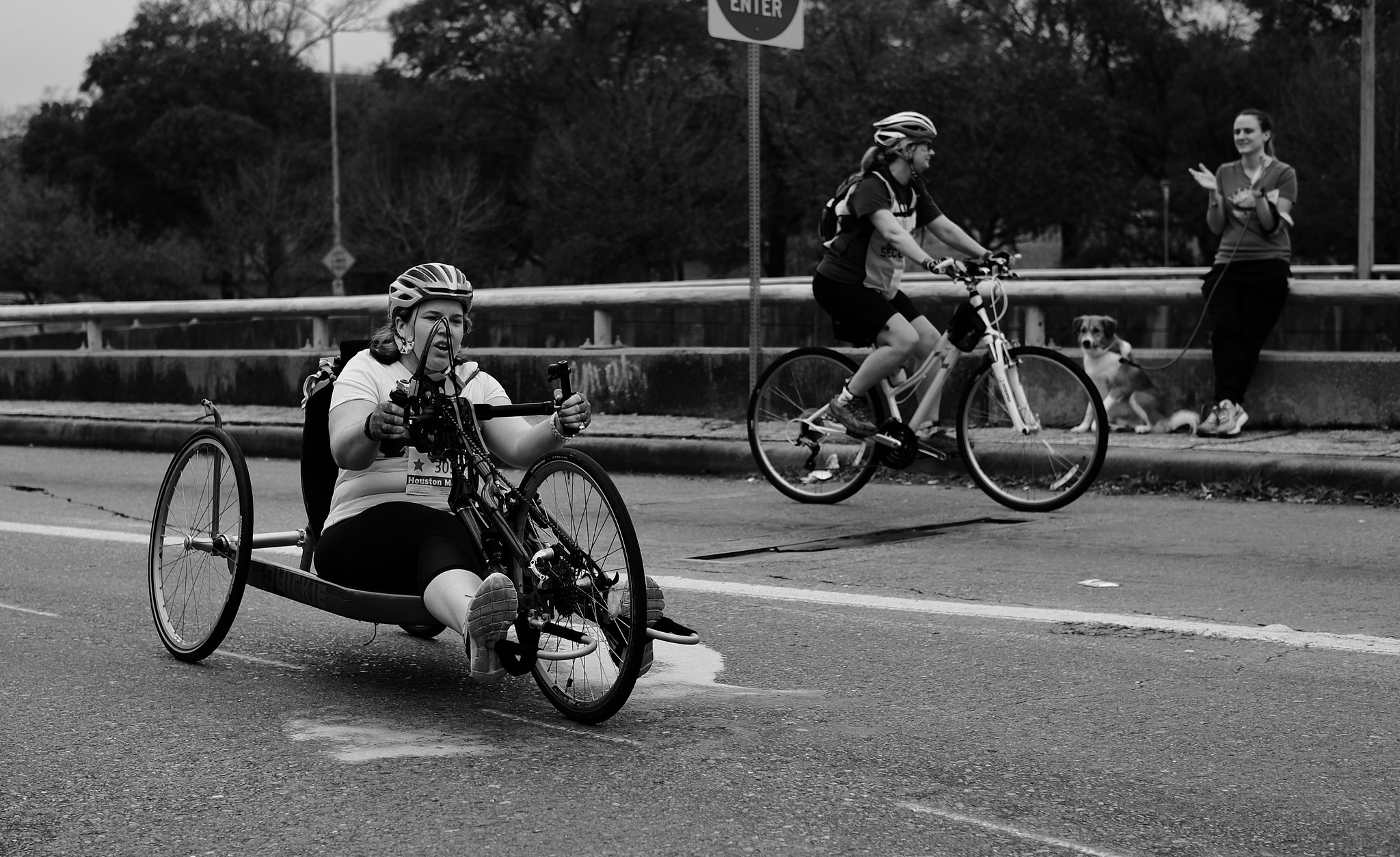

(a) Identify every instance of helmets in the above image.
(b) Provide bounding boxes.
[873,112,937,147]
[387,262,475,330]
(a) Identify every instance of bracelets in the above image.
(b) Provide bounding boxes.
[363,412,376,441]
[550,411,574,441]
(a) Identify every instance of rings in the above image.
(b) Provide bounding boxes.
[579,422,585,430]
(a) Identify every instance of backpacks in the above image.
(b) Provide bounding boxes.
[820,170,925,240]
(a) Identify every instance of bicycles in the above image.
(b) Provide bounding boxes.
[743,248,1111,512]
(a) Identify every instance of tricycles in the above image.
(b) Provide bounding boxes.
[146,310,703,728]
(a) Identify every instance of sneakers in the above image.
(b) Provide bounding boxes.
[823,386,876,433]
[915,421,975,461]
[608,572,665,679]
[1195,400,1249,438]
[465,573,519,684]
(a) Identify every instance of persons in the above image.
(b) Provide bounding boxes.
[312,263,663,685]
[1188,109,1298,439]
[814,111,1015,457]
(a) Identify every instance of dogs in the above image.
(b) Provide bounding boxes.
[1068,314,1202,438]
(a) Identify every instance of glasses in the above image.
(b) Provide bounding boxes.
[926,143,932,152]
